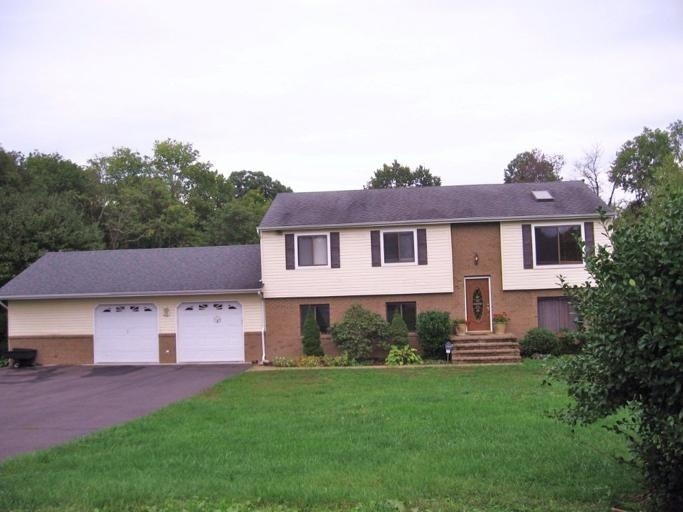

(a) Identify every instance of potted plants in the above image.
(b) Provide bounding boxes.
[453,319,467,336]
[448,319,456,334]
[494,312,511,334]
[563,328,586,346]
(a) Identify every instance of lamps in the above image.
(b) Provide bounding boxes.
[474,253,479,266]
